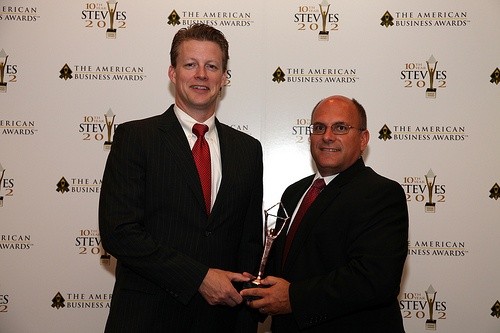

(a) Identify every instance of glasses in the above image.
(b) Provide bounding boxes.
[308,122,362,136]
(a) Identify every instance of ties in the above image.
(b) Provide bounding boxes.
[192,123,211,215]
[284,179,326,257]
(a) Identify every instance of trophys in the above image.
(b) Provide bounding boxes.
[231,200,290,323]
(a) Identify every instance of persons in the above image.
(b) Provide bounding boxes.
[97,23,264,332]
[239,94,409,333]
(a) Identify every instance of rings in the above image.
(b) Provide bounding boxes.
[260,307,266,314]
